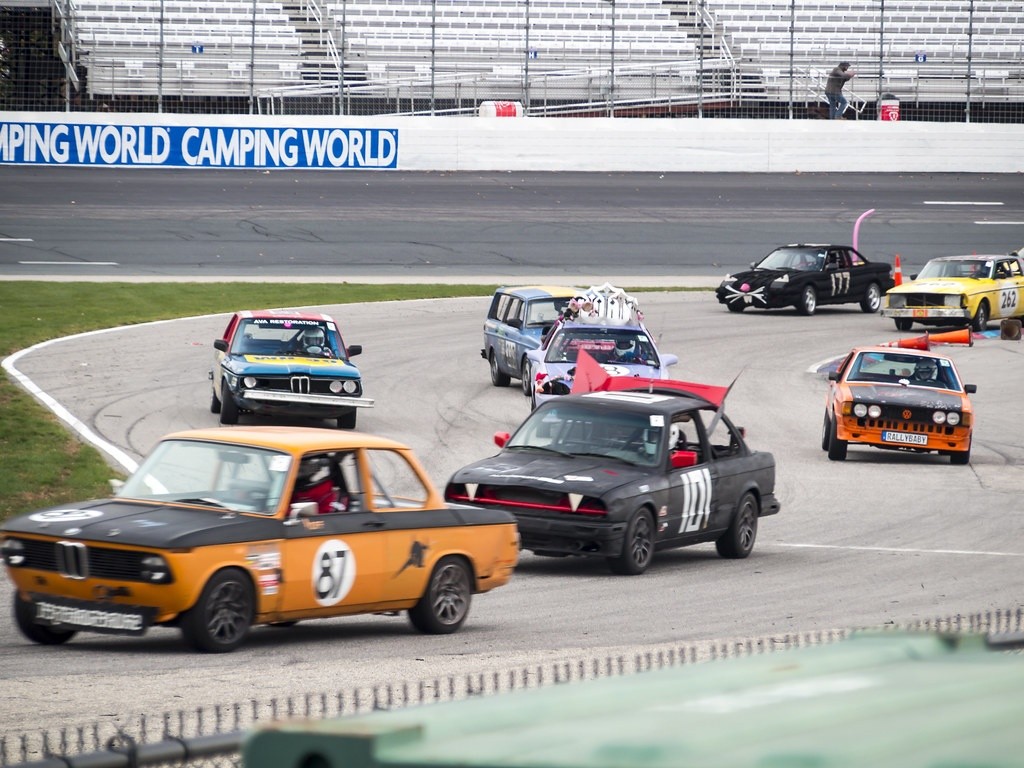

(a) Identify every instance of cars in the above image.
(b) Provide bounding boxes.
[207,311,376,429]
[715,244,894,316]
[444,373,778,575]
[526,315,678,437]
[822,346,976,464]
[0,425,517,653]
[880,255,1024,331]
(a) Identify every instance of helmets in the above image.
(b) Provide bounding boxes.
[298,454,333,489]
[817,253,830,265]
[643,423,680,455]
[914,358,938,381]
[614,339,635,356]
[302,328,326,348]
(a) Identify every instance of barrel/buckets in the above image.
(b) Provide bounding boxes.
[881,100,900,121]
[479,101,523,117]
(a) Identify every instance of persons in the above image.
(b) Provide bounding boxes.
[608,338,645,363]
[290,326,332,357]
[642,423,679,462]
[230,455,339,517]
[824,61,857,119]
[815,250,830,267]
[904,358,948,389]
[980,262,1001,275]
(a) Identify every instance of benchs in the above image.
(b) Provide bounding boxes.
[54,0,1024,102]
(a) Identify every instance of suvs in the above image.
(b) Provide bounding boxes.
[481,285,616,396]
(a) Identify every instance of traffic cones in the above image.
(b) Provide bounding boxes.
[1001,319,1023,340]
[877,331,931,352]
[969,247,979,273]
[926,327,973,347]
[892,251,904,289]
